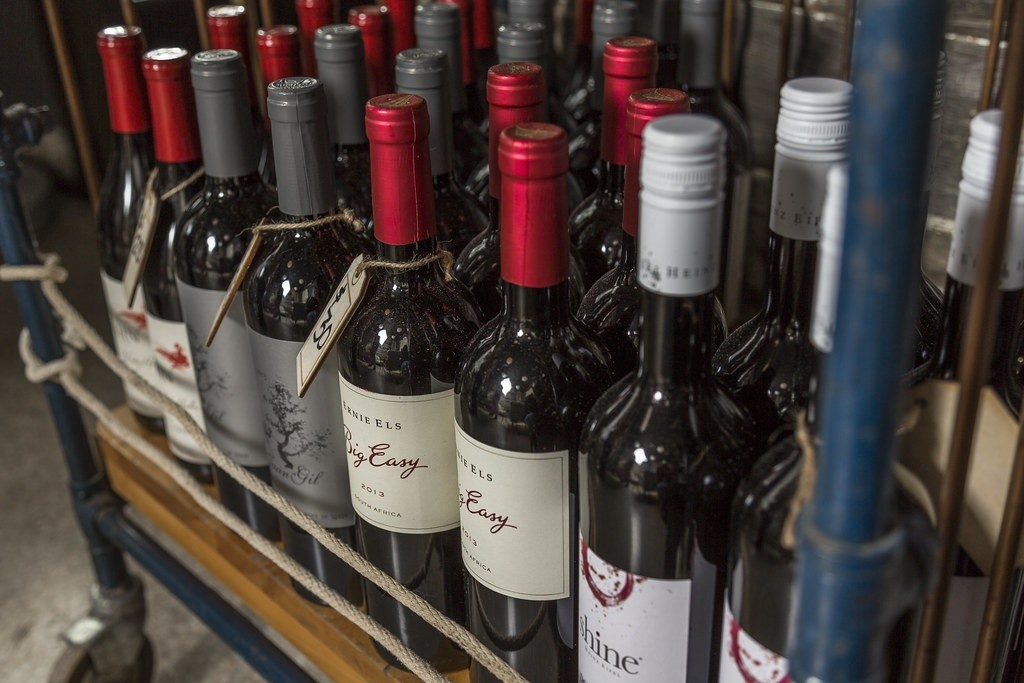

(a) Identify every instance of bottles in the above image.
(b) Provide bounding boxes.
[99,0,1024,683]
